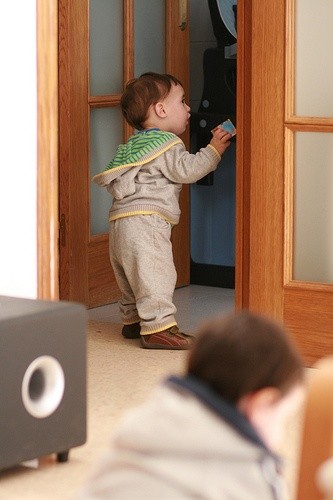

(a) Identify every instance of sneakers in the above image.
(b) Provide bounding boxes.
[122,322,142,340]
[142,326,196,350]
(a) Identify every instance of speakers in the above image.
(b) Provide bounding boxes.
[0,295,87,472]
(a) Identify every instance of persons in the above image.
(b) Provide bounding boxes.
[92,72,232,350]
[67,311,306,500]
